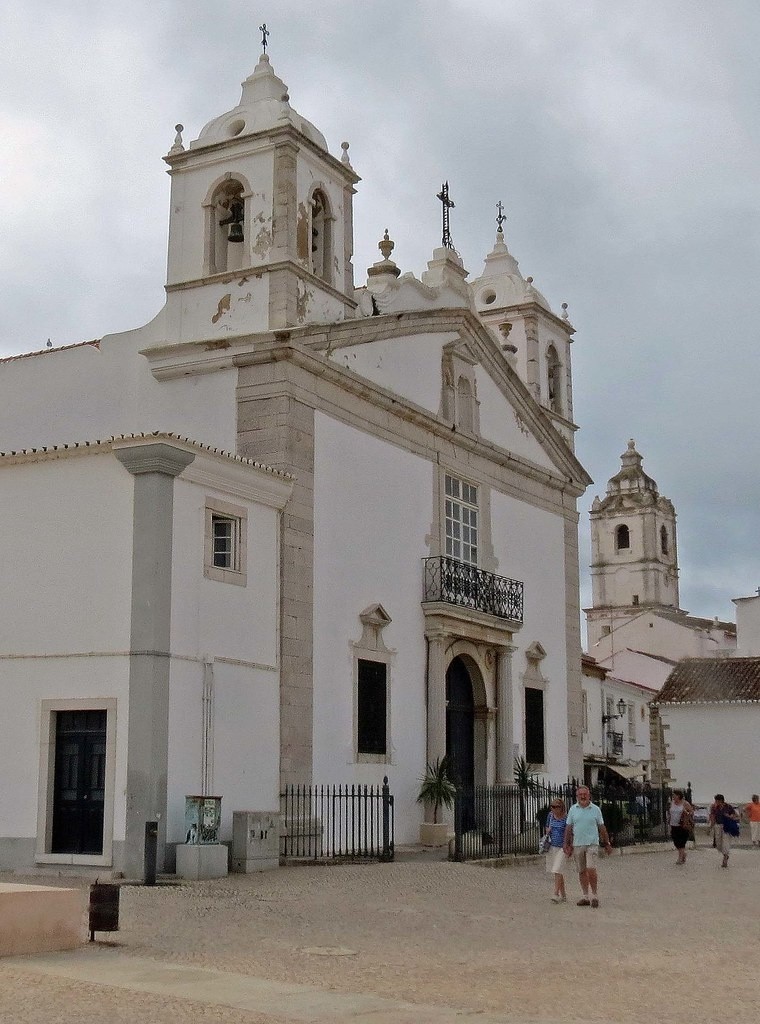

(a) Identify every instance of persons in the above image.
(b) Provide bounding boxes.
[743,795,760,847]
[707,794,741,868]
[670,790,694,865]
[563,786,612,908]
[546,798,573,903]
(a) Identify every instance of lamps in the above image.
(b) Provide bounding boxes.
[602,697,626,724]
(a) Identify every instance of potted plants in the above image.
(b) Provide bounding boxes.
[416,757,457,848]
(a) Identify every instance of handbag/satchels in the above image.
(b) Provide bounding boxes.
[722,817,740,837]
[679,811,694,831]
[539,835,552,855]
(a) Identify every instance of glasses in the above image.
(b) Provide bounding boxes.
[550,805,562,809]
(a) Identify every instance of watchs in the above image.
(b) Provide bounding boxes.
[605,842,611,845]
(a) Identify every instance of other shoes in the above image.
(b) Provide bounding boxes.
[577,899,590,906]
[676,852,687,865]
[550,896,567,904]
[591,899,599,908]
[721,855,729,868]
